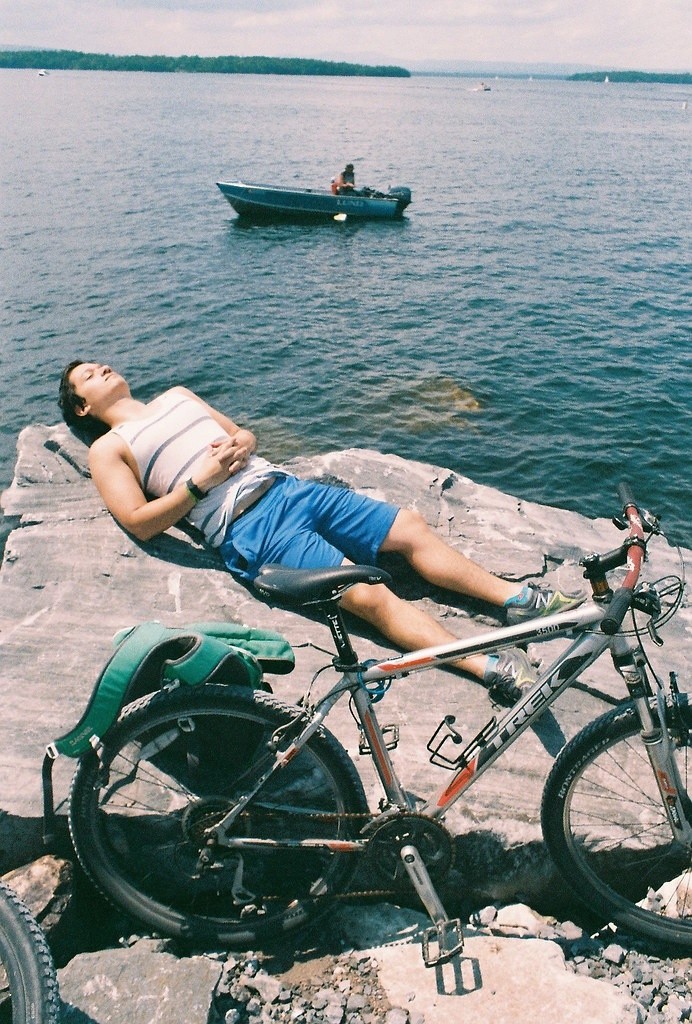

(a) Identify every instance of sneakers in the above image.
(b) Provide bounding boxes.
[506,581,588,628]
[486,648,540,707]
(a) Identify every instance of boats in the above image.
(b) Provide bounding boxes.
[211,177,414,223]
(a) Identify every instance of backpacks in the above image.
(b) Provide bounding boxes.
[54,619,296,781]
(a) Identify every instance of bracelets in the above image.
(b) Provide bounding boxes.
[185,477,208,504]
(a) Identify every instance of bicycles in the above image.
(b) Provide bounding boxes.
[61,477,691,972]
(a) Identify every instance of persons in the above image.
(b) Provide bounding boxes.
[58,360,589,722]
[335,164,355,196]
